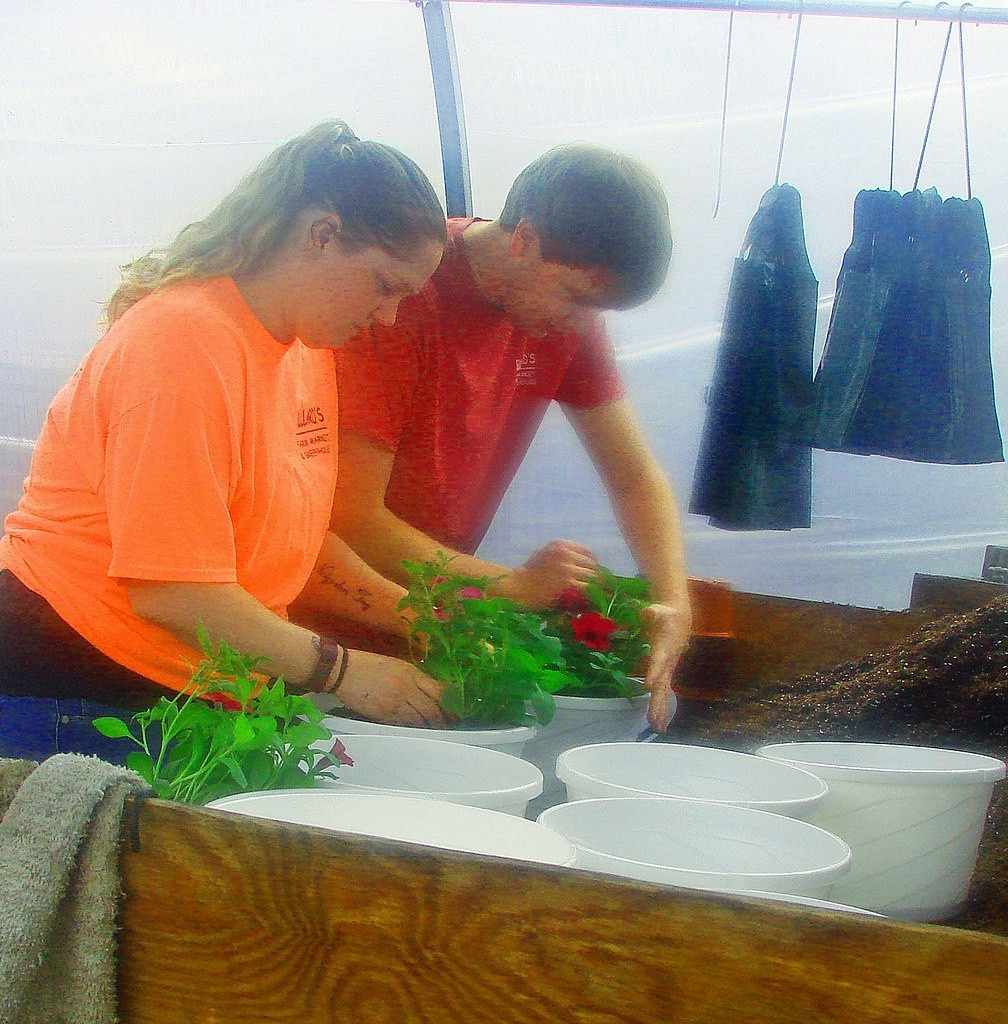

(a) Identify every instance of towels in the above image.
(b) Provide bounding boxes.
[0,749,148,1024]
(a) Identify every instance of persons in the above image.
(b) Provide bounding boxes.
[339,137,694,736]
[1,116,495,785]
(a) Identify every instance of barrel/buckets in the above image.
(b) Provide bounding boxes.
[201,734,1006,935]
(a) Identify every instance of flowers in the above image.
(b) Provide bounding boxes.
[394,548,660,726]
[90,618,354,808]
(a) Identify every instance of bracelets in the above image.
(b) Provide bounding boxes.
[296,636,350,694]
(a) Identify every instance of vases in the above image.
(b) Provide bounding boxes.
[554,743,826,825]
[268,735,545,820]
[204,787,582,870]
[755,739,1007,925]
[295,687,535,760]
[523,678,653,822]
[534,798,851,904]
